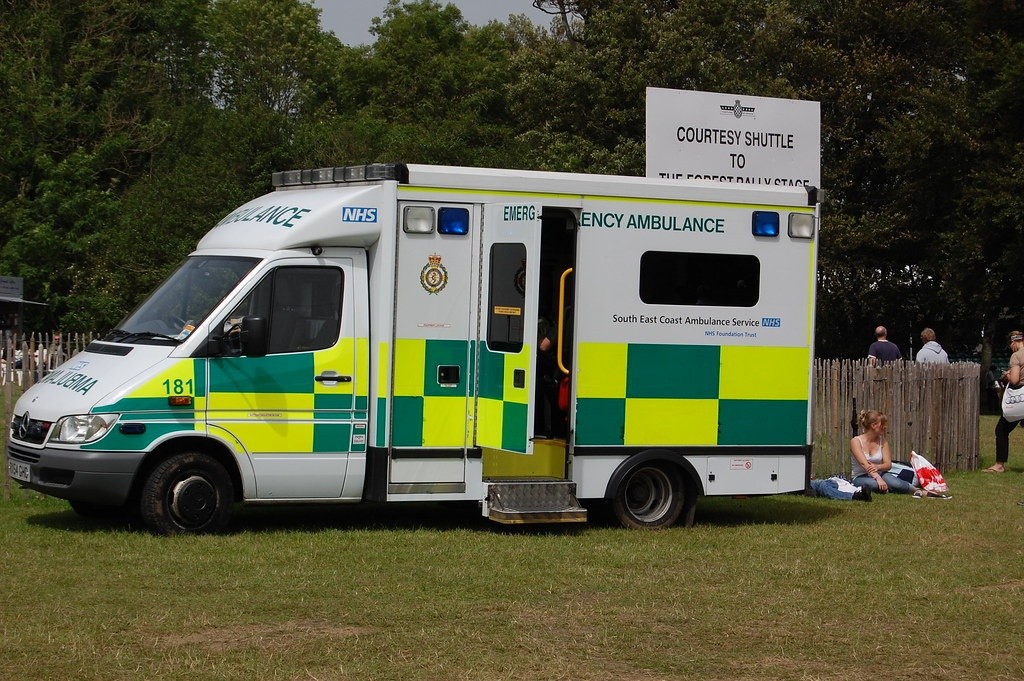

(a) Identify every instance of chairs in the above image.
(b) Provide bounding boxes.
[268,311,306,352]
[296,319,339,350]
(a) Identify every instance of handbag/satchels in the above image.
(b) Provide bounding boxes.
[1001,386,1024,422]
[909,450,949,494]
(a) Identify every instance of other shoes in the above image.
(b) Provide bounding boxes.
[912,490,923,498]
[926,492,953,500]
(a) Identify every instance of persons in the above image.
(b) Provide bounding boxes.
[810,473,872,502]
[915,328,950,364]
[0,332,63,369]
[981,331,1024,473]
[538,303,574,366]
[867,326,903,365]
[849,410,953,500]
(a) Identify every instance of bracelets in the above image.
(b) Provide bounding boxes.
[1005,372,1010,376]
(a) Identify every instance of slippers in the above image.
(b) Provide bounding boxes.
[981,468,1005,473]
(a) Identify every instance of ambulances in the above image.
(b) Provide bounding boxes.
[10,163,824,534]
